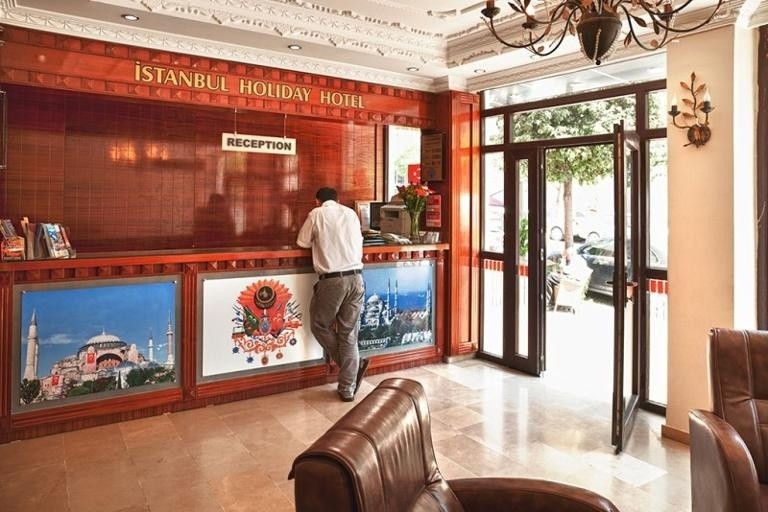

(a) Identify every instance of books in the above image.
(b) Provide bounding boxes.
[1,217,71,260]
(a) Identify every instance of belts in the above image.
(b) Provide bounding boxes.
[318,268,362,278]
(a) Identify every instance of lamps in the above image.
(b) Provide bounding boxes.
[668,70,718,148]
[480,1,726,65]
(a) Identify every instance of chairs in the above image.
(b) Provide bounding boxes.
[286,376,618,512]
[685,328,768,512]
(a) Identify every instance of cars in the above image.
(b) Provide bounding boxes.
[546,208,662,297]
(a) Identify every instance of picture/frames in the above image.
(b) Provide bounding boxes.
[354,200,372,230]
[419,130,446,183]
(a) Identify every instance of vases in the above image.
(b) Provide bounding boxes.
[408,209,424,244]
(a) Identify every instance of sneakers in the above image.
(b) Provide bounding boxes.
[337,357,370,401]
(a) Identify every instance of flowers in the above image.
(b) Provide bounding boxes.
[397,181,435,234]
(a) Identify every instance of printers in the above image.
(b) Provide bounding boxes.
[379,200,412,238]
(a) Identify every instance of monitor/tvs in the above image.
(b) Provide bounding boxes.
[370,202,389,231]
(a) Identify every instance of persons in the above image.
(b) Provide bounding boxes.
[296,186,365,401]
